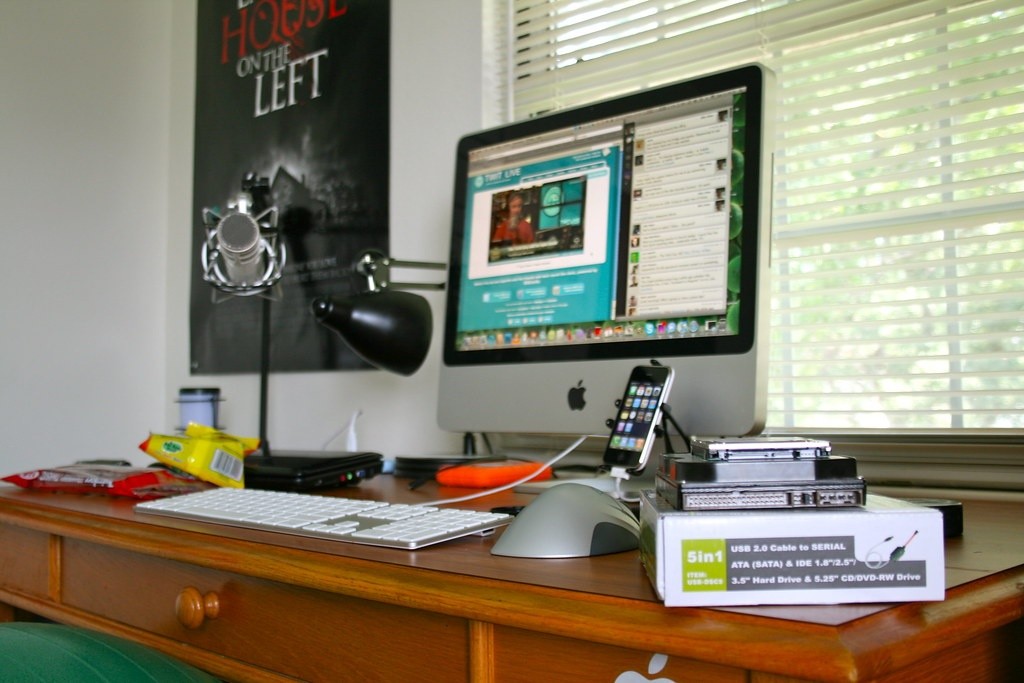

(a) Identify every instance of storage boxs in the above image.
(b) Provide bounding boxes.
[637,486,945,608]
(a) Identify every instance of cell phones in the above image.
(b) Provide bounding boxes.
[601,365,675,470]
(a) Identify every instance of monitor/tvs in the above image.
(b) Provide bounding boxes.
[435,60,778,501]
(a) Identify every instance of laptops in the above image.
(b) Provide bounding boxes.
[241,453,385,491]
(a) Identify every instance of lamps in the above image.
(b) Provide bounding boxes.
[197,169,445,482]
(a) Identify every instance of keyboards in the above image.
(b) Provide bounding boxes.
[136,486,512,549]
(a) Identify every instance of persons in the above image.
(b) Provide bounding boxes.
[493,194,533,246]
[716,111,727,212]
[632,238,640,247]
[629,267,638,316]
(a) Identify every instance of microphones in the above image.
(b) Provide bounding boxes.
[215,213,266,283]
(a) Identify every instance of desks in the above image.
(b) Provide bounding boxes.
[0,471,1024,683]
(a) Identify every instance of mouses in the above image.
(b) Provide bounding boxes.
[490,482,639,557]
